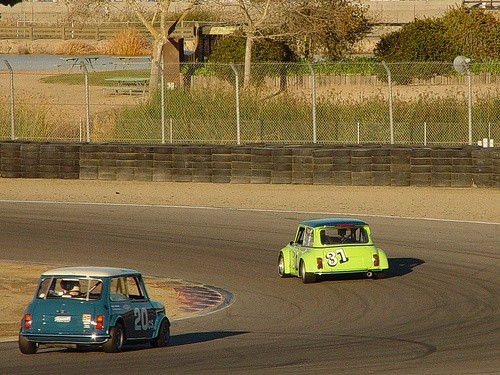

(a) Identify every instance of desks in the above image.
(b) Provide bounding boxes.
[105,77,150,95]
[112,56,151,70]
[61,57,99,72]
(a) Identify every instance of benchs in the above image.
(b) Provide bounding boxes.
[102,86,147,96]
[112,62,152,71]
[58,62,97,72]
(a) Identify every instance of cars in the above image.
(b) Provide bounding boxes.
[277,217,389,284]
[19,266,170,353]
